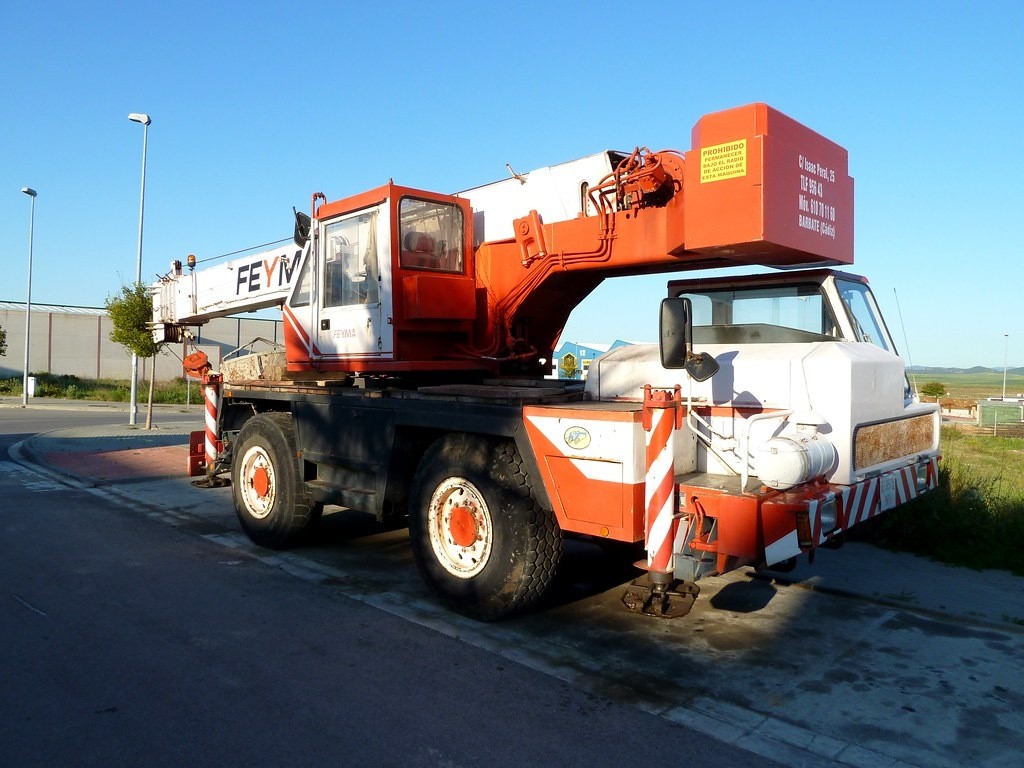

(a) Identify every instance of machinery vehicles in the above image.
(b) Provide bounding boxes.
[146,103,943,624]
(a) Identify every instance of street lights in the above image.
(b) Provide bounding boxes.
[128,112,152,425]
[22,187,37,406]
[1003,333,1008,398]
